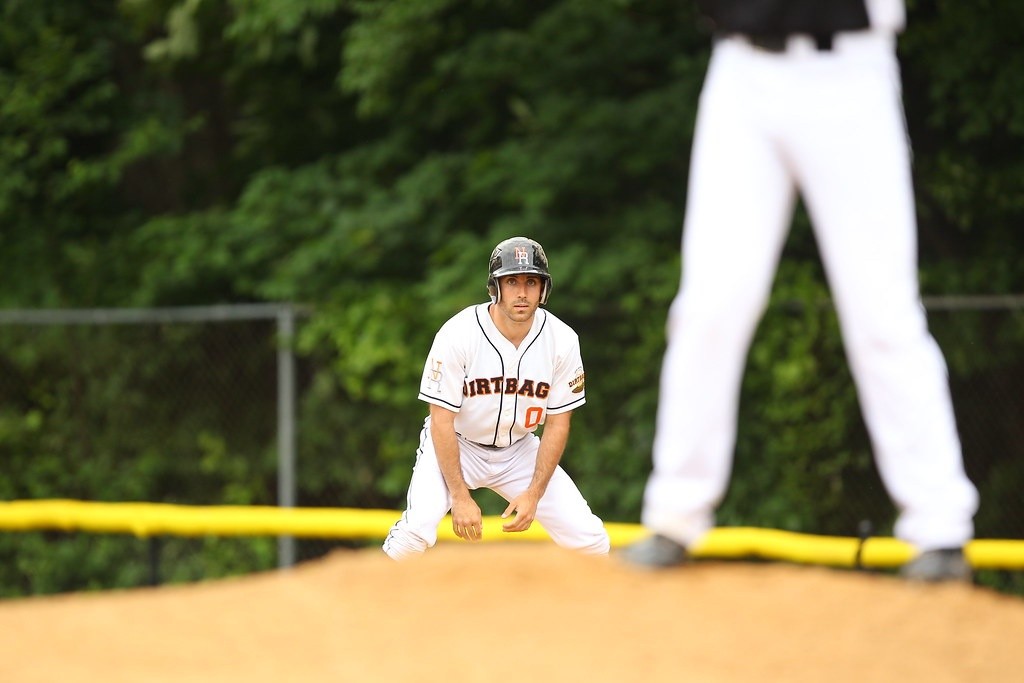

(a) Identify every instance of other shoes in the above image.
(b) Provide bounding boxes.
[618,533,691,570]
[905,548,975,583]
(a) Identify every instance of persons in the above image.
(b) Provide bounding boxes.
[379,235,612,559]
[615,0,982,584]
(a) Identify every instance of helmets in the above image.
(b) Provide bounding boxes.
[486,238,553,309]
